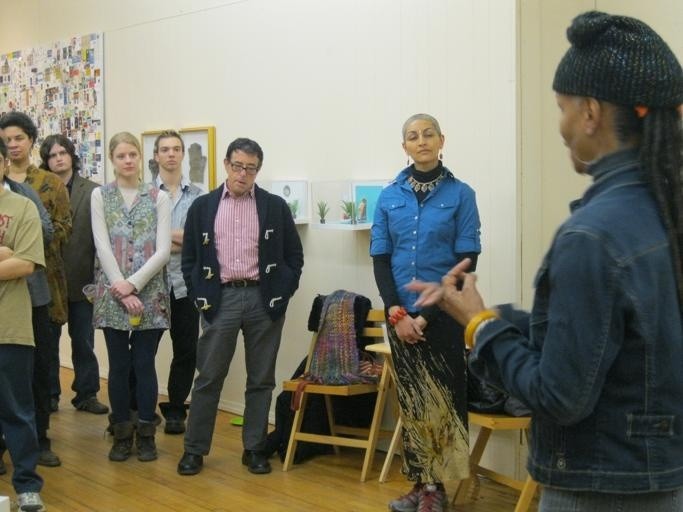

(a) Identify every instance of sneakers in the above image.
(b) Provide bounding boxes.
[389,482,424,512]
[16,492,45,512]
[417,484,445,511]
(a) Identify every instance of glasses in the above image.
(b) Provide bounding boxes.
[229,160,259,175]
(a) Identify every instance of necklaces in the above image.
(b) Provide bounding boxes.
[406,173,445,193]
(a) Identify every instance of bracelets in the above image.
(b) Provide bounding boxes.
[388,307,409,325]
[464,310,496,349]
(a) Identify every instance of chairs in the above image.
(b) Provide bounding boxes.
[452,412,543,512]
[283,309,391,482]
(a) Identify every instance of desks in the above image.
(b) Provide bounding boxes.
[365,342,410,482]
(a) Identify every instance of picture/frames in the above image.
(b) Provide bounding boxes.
[138,126,216,192]
[271,178,311,224]
[0,31,105,185]
[352,180,388,224]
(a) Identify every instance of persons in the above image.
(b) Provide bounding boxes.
[178,138,304,473]
[0,140,48,511]
[90,132,171,461]
[0,138,62,468]
[152,132,207,435]
[42,134,102,412]
[369,114,480,512]
[441,12,683,512]
[2,111,69,411]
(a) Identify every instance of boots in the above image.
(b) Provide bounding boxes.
[137,421,161,460]
[110,422,134,460]
[162,402,187,433]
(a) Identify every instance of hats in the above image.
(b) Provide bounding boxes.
[552,11,683,107]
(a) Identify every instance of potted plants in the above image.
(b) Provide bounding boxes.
[340,200,357,224]
[315,200,329,224]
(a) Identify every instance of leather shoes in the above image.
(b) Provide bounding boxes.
[177,453,204,475]
[37,449,61,467]
[73,397,108,413]
[243,450,270,473]
